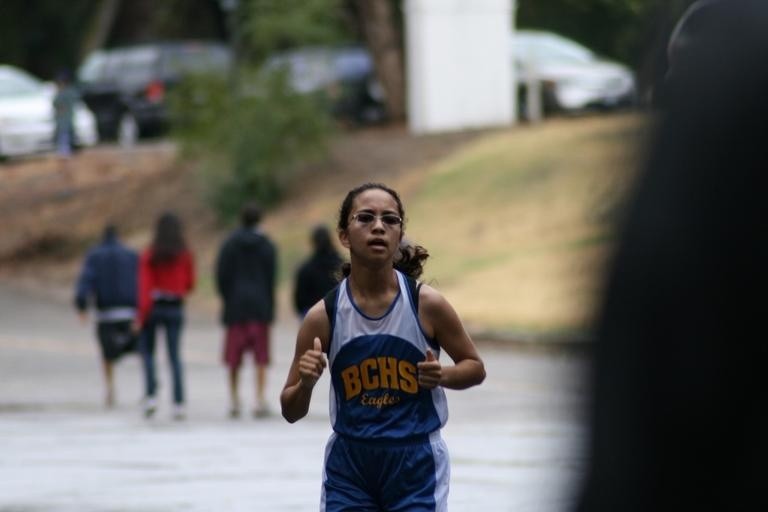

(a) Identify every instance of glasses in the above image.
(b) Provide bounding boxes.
[346,214,404,226]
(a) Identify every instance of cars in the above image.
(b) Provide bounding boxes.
[512,28,636,123]
[1,65,98,164]
[248,44,380,133]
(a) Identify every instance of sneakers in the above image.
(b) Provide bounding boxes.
[228,407,274,420]
[141,395,189,421]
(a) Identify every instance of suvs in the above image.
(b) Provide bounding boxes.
[80,40,239,145]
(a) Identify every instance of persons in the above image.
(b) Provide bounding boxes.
[281,180,485,512]
